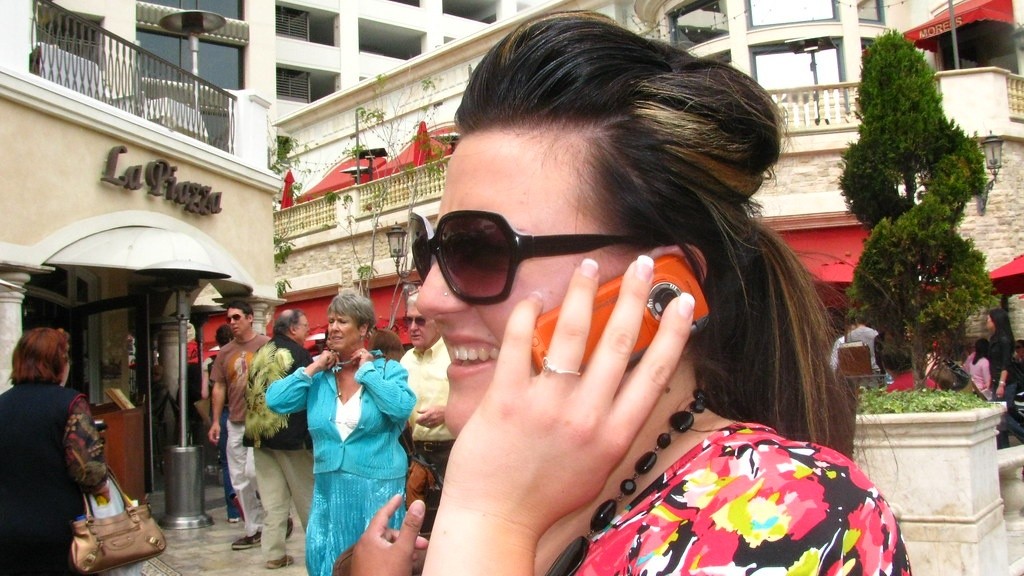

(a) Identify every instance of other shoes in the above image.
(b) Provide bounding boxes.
[266,554,294,568]
[234,496,246,522]
[229,518,239,523]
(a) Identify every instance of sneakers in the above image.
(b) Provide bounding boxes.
[285,517,293,538]
[231,532,262,549]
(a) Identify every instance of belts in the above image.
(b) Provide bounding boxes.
[414,441,454,452]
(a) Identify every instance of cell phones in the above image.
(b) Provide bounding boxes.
[525,253,709,376]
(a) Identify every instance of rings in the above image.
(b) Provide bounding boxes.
[542,357,580,376]
[360,352,362,356]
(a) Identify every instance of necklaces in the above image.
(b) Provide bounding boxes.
[544,388,707,576]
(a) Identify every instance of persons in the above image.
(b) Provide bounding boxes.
[410,9,913,576]
[1014,340,1024,362]
[832,312,878,381]
[0,328,108,576]
[987,308,1024,448]
[963,339,991,390]
[202,289,455,576]
[882,342,940,392]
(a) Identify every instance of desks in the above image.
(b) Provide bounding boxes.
[30,42,105,101]
[144,97,209,143]
[158,10,227,138]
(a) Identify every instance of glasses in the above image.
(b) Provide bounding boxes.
[403,316,426,327]
[226,313,249,324]
[407,210,666,305]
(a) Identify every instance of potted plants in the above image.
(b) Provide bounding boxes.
[850,383,1008,523]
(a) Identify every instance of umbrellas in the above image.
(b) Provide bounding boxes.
[414,121,432,169]
[989,254,1024,294]
[281,169,295,210]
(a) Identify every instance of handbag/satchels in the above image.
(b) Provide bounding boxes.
[405,460,436,510]
[68,464,166,574]
[89,477,143,576]
[931,358,970,392]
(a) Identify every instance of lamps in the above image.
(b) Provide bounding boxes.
[385,221,416,278]
[978,130,1005,217]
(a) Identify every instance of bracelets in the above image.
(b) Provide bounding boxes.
[999,381,1006,385]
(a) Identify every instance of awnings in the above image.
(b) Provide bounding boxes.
[188,281,415,363]
[905,0,1014,53]
[769,227,870,309]
[299,157,386,203]
[373,137,451,179]
